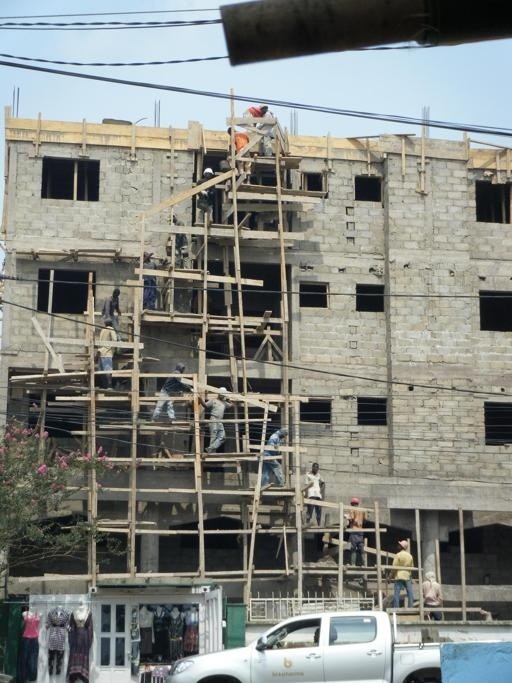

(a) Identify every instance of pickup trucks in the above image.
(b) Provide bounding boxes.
[166,609,511,681]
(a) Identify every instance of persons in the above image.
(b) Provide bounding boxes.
[197,387,233,453]
[244,106,268,127]
[196,168,214,225]
[313,628,319,646]
[344,497,368,567]
[170,607,184,658]
[183,607,199,652]
[143,252,159,310]
[261,428,289,487]
[389,540,413,608]
[227,127,251,184]
[154,607,169,656]
[151,363,193,423]
[45,606,70,675]
[423,572,443,621]
[139,606,152,654]
[17,607,43,682]
[68,605,93,683]
[98,318,117,390]
[304,463,325,527]
[102,288,121,341]
[173,215,188,268]
